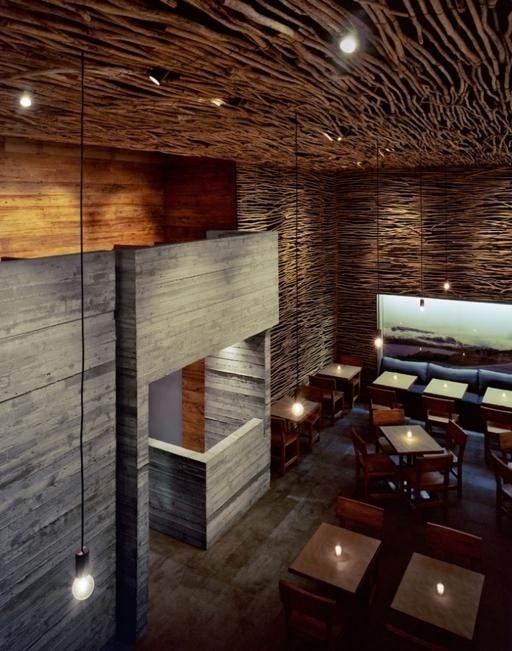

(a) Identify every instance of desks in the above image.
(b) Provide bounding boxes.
[387,551,487,643]
[288,521,381,599]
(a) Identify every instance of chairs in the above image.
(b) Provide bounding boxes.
[425,520,481,567]
[336,494,383,540]
[276,579,339,651]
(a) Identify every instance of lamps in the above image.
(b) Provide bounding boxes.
[411,147,425,308]
[288,115,311,416]
[70,50,97,603]
[437,154,452,291]
[370,130,384,348]
